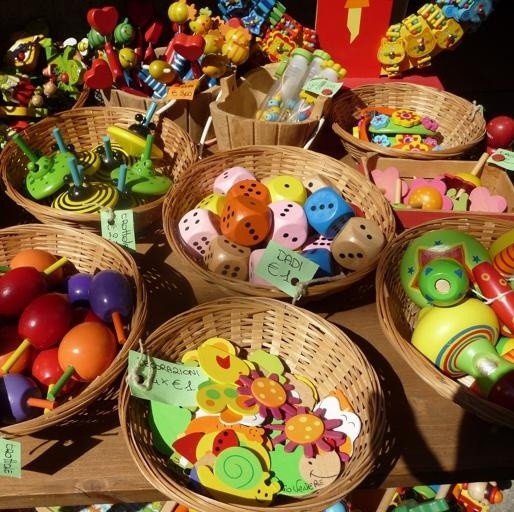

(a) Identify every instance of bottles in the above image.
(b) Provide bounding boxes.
[255,46,348,122]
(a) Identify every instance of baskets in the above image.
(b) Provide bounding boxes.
[161,145,396,305]
[329,83,487,166]
[118,298,386,512]
[0,106,198,236]
[1,225,149,438]
[376,216,513,430]
